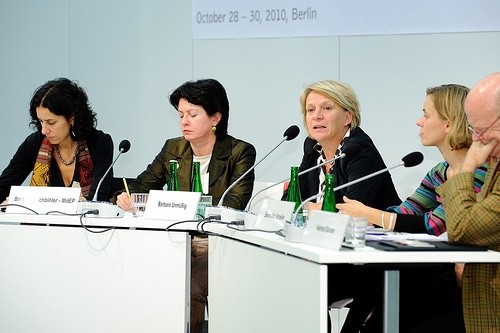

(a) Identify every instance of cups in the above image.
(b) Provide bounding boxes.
[130,194,147,217]
[343,216,368,247]
[194,196,212,218]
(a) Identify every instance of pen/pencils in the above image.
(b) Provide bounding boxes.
[365,233,388,235]
[121,177,130,201]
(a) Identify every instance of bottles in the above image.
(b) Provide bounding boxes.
[288,167,304,228]
[321,174,337,213]
[191,161,203,194]
[168,159,179,191]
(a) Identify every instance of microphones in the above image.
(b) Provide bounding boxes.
[77,140,131,218]
[204,125,424,243]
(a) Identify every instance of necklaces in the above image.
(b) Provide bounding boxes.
[54,145,77,165]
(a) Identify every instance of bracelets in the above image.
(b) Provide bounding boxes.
[389,212,395,231]
[381,210,386,231]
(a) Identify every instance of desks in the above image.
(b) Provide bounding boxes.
[0,212,500,333]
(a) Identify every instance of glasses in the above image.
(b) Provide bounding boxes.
[468,117,500,138]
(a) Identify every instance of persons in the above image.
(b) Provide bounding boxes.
[0,78,114,203]
[336,73,500,333]
[281,79,401,333]
[110,79,256,333]
